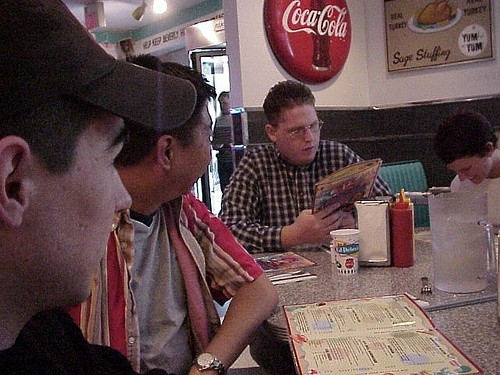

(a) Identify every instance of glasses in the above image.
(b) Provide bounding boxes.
[275,118,324,138]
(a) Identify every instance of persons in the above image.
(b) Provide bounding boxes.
[0,0,197,375]
[429,108,500,235]
[64,53,279,375]
[212,91,235,192]
[218,80,392,375]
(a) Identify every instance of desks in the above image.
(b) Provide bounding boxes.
[241,225,500,375]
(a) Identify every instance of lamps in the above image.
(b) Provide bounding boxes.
[132,0,150,22]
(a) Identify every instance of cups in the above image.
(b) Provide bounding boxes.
[330,228,362,274]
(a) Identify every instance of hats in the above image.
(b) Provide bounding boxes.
[0,0,197,131]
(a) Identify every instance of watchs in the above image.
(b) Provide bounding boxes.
[193,352,225,375]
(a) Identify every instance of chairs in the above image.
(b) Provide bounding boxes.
[377,159,430,232]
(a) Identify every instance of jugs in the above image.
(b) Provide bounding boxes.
[427,191,496,294]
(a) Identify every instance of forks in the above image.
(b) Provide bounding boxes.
[268,265,310,277]
[421,277,432,294]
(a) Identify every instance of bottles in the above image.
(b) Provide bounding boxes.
[390,188,416,267]
[312,0,332,72]
